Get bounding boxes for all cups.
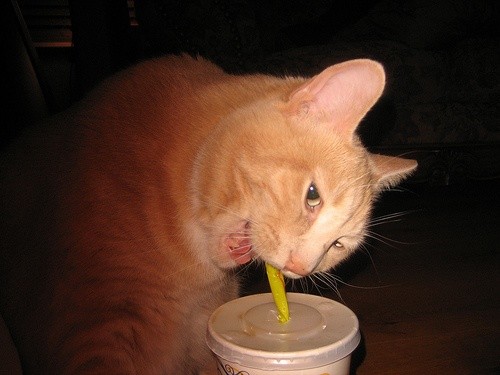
[206,292,361,375]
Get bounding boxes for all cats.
[0,53,419,375]
